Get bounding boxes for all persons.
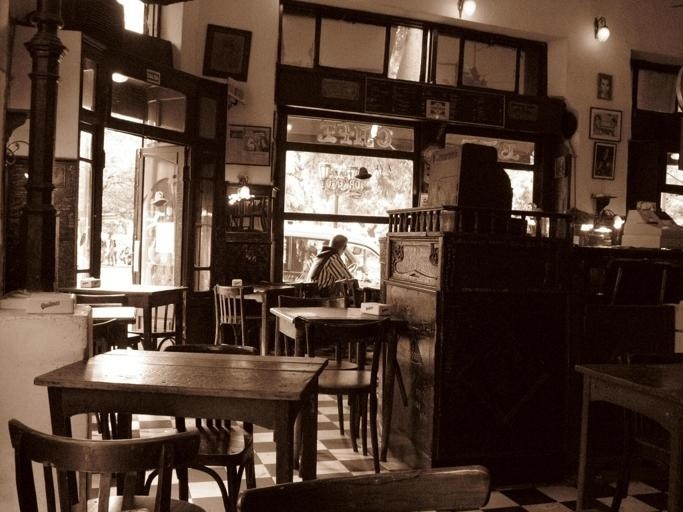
[79,232,132,267]
[594,114,616,137]
[309,235,354,305]
[598,76,613,100]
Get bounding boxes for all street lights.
[317,159,363,229]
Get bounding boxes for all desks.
[33,350,329,495]
[571,358,682,511]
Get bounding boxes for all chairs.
[2,280,408,474]
[144,346,256,511]
[9,418,204,511]
[235,465,491,511]
[578,252,673,511]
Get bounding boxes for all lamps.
[456,1,477,21]
[593,15,611,42]
[577,196,625,237]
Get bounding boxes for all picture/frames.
[586,105,623,144]
[201,22,253,84]
[590,138,616,182]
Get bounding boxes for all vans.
[282,224,381,286]
